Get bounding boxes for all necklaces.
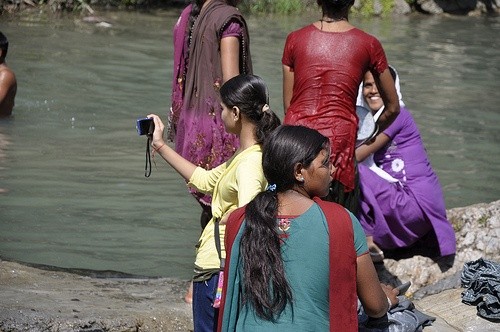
[278,197,304,207]
[320,18,347,30]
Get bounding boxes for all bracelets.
[385,296,391,312]
[156,143,164,151]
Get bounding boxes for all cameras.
[136,117,155,136]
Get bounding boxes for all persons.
[168,0,253,302]
[354,65,456,259]
[282,0,400,220]
[146,74,282,332]
[0,30,16,118]
[216,124,400,332]
